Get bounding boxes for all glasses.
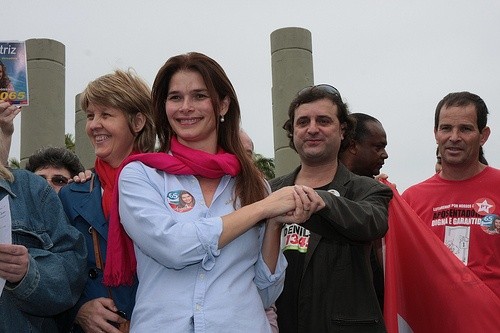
[37,174,69,186]
[296,84,343,102]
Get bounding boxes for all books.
[0,40,29,106]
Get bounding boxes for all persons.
[24,52,307,333]
[178,190,195,208]
[487,216,500,233]
[266,84,500,333]
[0,59,15,105]
[0,99,88,333]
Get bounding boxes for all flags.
[379,177,500,333]
